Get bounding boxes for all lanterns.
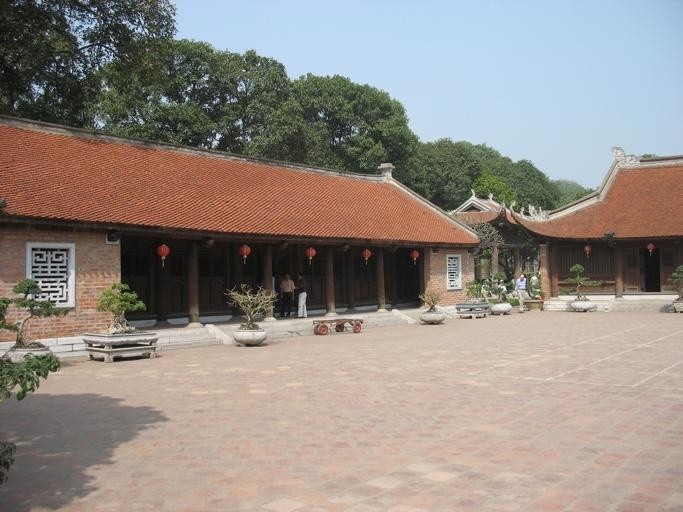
[238,244,251,264]
[410,250,419,265]
[583,245,591,257]
[156,243,169,268]
[304,247,316,266]
[361,248,371,265]
[646,242,654,256]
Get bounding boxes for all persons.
[529,274,538,297]
[292,272,308,318]
[480,278,493,304]
[514,274,528,312]
[278,272,295,317]
[488,274,516,303]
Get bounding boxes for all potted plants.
[0,279,158,366]
[418,287,447,324]
[454,272,546,318]
[662,264,683,314]
[557,262,606,313]
[222,282,280,348]
